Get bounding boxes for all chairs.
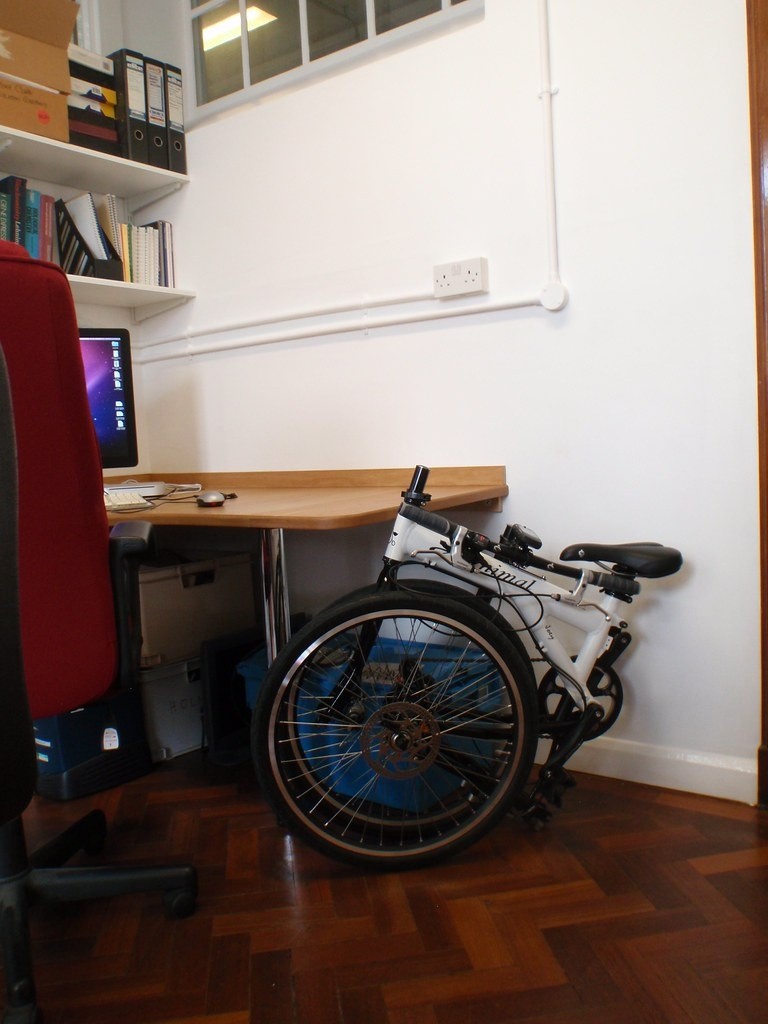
[0,238,198,1024]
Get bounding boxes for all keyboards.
[102,491,155,511]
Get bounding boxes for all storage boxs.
[0,0,79,142]
[140,657,212,761]
[139,546,254,669]
[238,633,499,813]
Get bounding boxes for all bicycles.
[251,467,685,872]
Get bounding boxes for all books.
[0,41,190,290]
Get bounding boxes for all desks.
[97,466,509,783]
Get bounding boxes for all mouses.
[197,492,225,507]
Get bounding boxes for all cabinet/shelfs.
[0,124,194,324]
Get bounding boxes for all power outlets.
[432,258,458,299]
[458,260,489,298]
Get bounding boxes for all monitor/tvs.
[78,328,138,469]
